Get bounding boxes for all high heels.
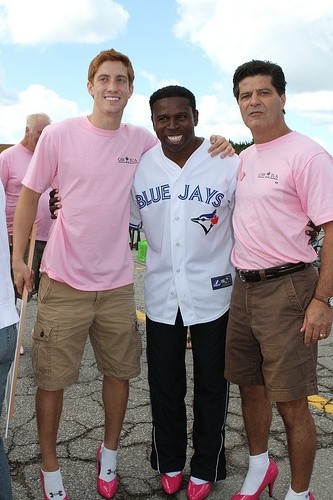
[96,443,118,498]
[39,469,68,500]
[308,491,315,500]
[231,456,278,500]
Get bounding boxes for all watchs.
[313,295,333,308]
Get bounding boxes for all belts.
[235,262,305,282]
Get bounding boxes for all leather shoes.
[187,478,211,500]
[162,473,182,495]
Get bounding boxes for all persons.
[12,48,236,500]
[224,60,333,500]
[0,177,19,500]
[0,114,55,354]
[49,86,321,500]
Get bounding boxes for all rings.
[319,334,326,338]
